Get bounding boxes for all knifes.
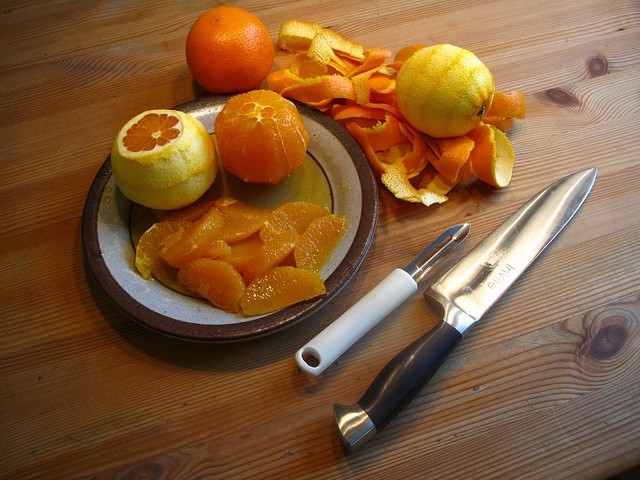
[334,165,598,455]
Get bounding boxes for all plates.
[83,91,377,343]
